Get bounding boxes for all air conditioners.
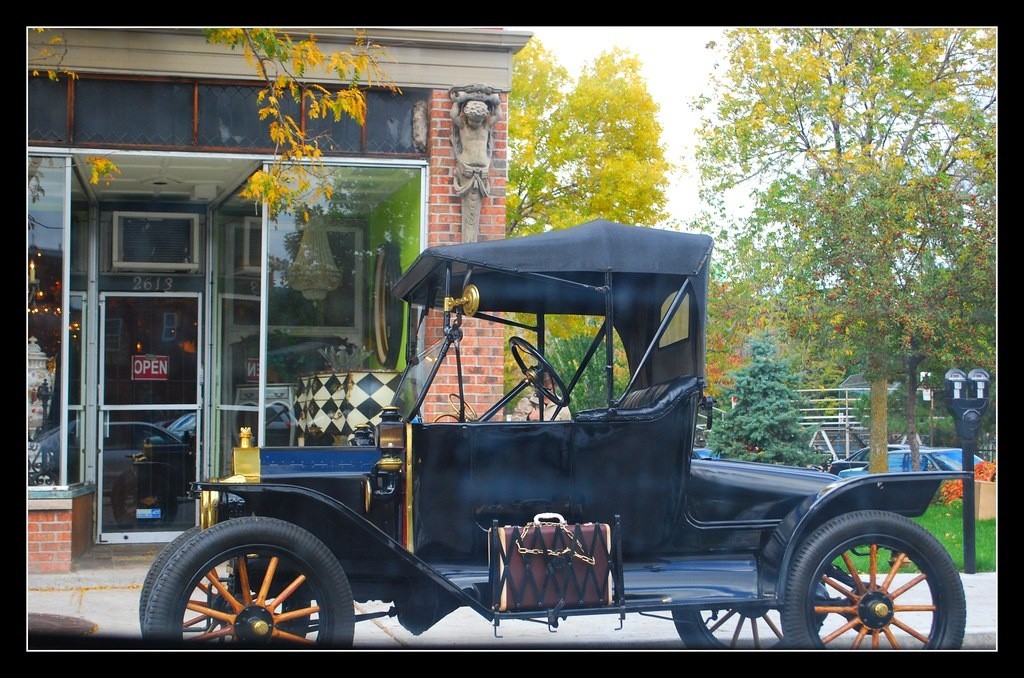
[224,215,262,276]
[111,211,199,274]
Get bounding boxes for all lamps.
[291,212,340,307]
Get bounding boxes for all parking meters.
[944,368,991,575]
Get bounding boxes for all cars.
[26,420,198,531]
[838,447,987,480]
[692,447,720,459]
[827,442,928,477]
[145,405,300,452]
[136,219,967,648]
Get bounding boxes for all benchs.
[572,374,699,422]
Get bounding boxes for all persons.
[510,364,571,421]
